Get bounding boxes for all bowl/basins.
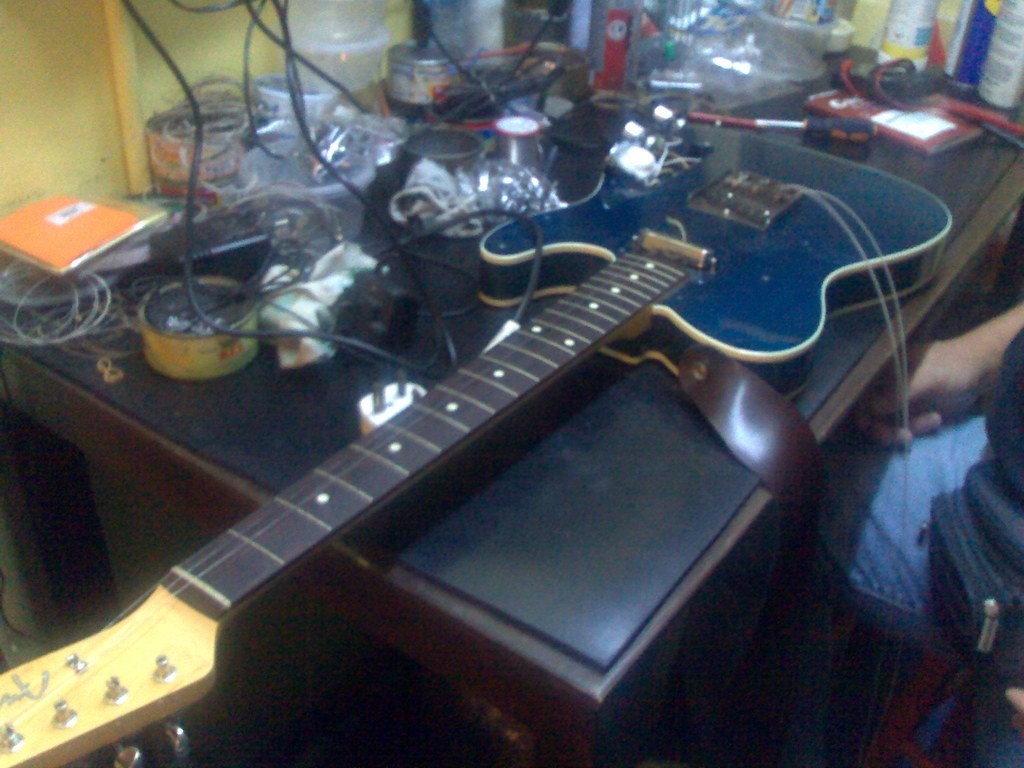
[280,0,391,92]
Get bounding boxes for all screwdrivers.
[756,111,877,145]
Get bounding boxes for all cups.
[406,129,483,181]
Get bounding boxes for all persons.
[699,308,1024,737]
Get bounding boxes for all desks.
[0,74,1021,768]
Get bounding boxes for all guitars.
[2,127,955,767]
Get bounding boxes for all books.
[0,193,168,275]
[805,88,985,157]
